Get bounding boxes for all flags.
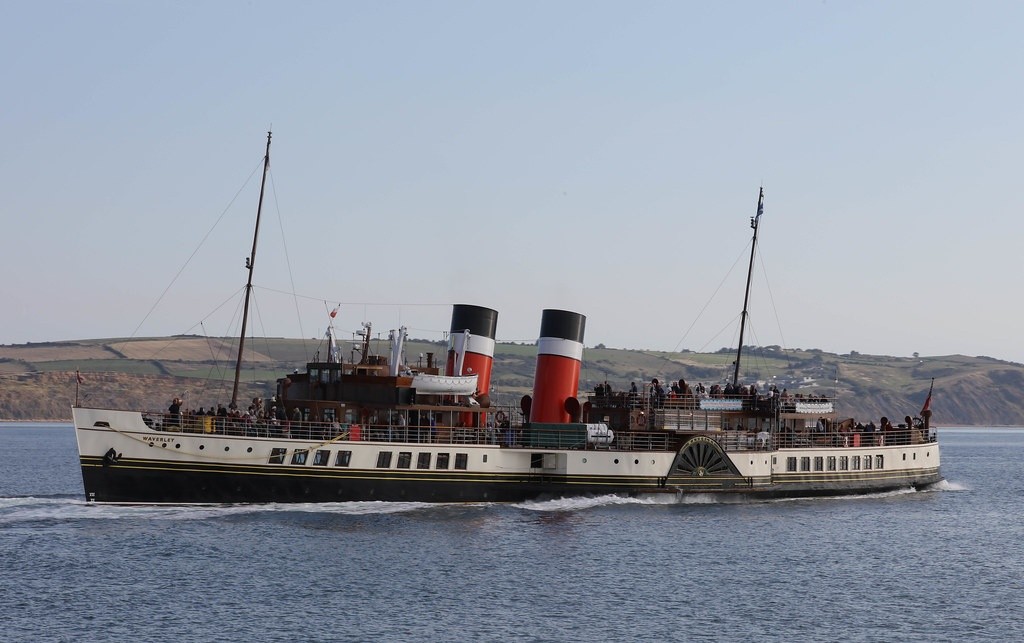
[756,192,765,217]
[919,388,932,417]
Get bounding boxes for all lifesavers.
[637,413,646,426]
[496,411,505,423]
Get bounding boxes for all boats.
[396,366,479,397]
[70,122,947,510]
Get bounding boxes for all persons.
[168,398,180,432]
[594,379,829,409]
[292,407,302,439]
[314,415,348,440]
[838,421,876,447]
[816,419,824,432]
[184,404,282,437]
[397,414,405,439]
[495,416,512,448]
[420,414,429,444]
